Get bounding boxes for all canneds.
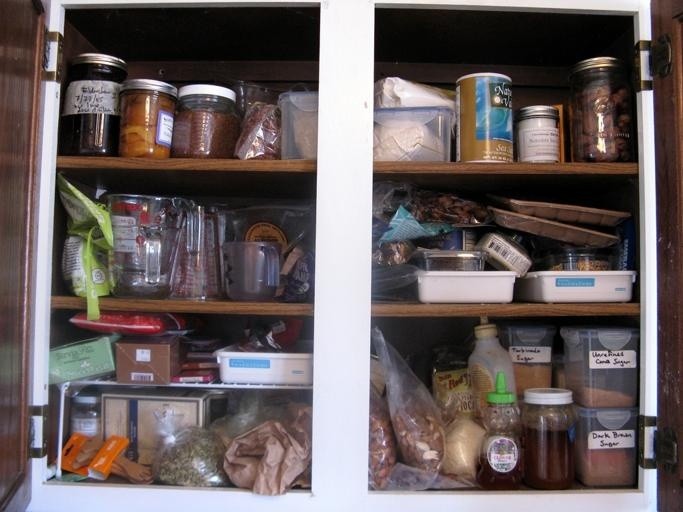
[455,71,514,165]
[58,53,127,157]
[70,395,102,439]
[521,387,576,490]
[117,78,177,161]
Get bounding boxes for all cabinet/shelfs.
[31,0,657,511]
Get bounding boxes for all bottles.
[467,324,518,426]
[171,204,221,297]
[477,370,529,489]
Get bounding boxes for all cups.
[220,237,284,302]
[102,192,199,300]
